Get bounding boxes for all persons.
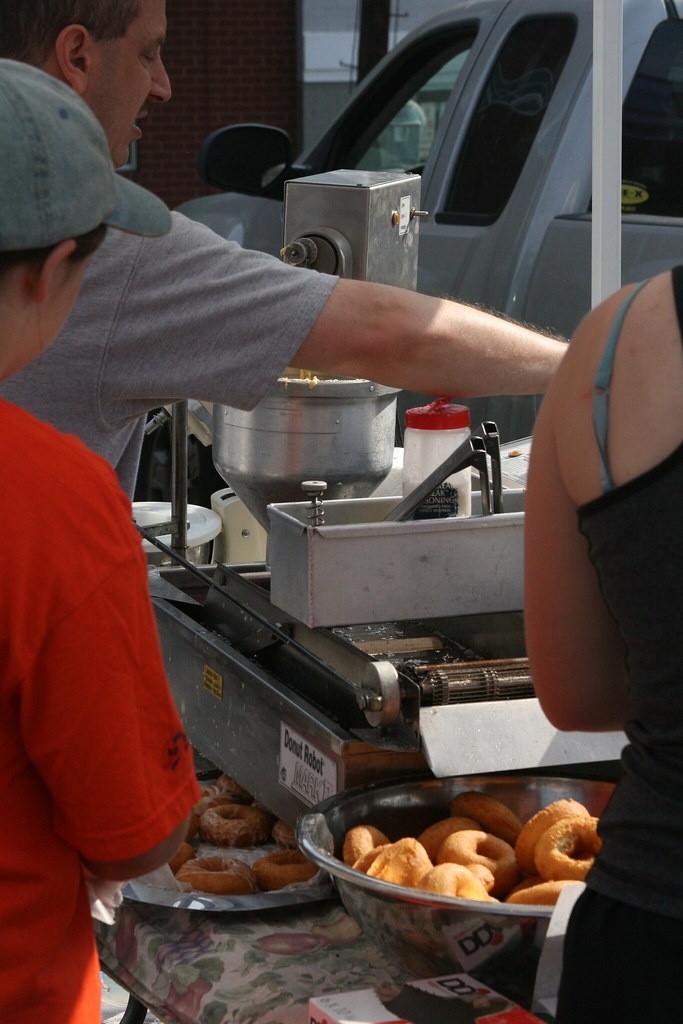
[0,57,200,1024]
[0,0,568,503]
[523,263,683,1024]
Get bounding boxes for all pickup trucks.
[133,0,683,506]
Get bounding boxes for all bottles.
[402,398,472,520]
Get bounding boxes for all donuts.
[169,774,319,896]
[344,792,599,906]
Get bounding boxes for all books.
[308,973,546,1024]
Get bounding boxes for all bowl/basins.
[293,774,615,982]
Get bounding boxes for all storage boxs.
[307,977,550,1024]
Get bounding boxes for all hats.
[0,57,173,251]
